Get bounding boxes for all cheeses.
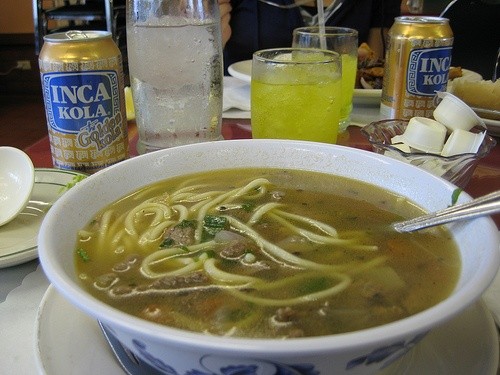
[451,77,500,110]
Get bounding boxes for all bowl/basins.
[36,137,500,375]
[360,118,496,191]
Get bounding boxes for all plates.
[470,106,500,135]
[225,57,484,105]
[0,166,92,269]
[36,282,500,375]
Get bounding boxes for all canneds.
[39,30,130,174]
[380,16,452,120]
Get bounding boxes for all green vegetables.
[80,185,358,321]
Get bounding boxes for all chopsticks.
[395,188,500,231]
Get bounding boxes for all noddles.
[77,167,461,339]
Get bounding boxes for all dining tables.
[0,85,500,375]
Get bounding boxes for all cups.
[291,26,358,134]
[125,1,225,154]
[248,47,341,144]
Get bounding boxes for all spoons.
[0,146,34,226]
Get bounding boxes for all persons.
[217,0,403,76]
